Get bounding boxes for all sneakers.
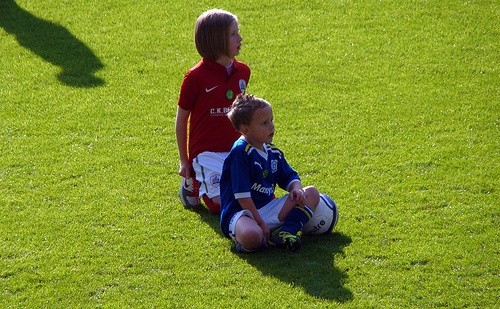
[271,230,302,252]
[179,175,200,207]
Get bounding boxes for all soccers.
[301,194,340,235]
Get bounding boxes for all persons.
[218,95,319,253]
[175,9,275,214]
